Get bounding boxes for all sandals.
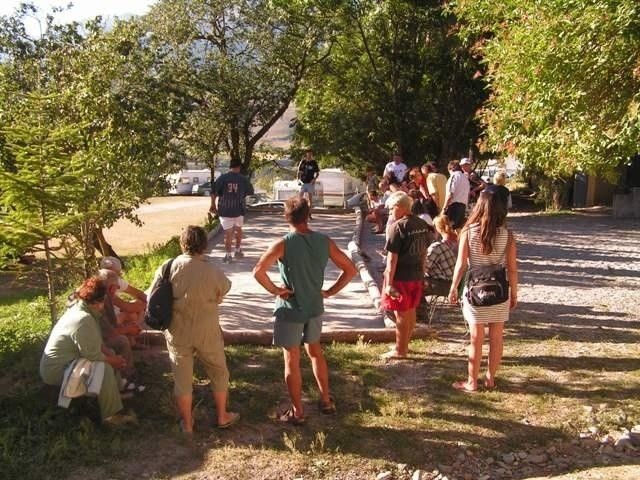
[119,381,145,392]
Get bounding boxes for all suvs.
[474,168,493,186]
[246,193,272,205]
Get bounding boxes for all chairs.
[427,262,470,340]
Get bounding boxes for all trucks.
[167,169,222,196]
[273,181,323,206]
[312,169,352,207]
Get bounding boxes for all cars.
[190,181,216,196]
[503,168,516,179]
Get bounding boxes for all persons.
[297,150,320,220]
[209,158,254,263]
[252,197,357,425]
[365,153,516,394]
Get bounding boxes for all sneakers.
[321,394,337,414]
[224,256,231,263]
[235,249,243,256]
[280,408,304,424]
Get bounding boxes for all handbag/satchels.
[144,278,172,329]
[465,264,509,305]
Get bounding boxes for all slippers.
[453,382,477,393]
[218,411,241,429]
[483,379,498,392]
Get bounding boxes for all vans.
[248,200,286,215]
